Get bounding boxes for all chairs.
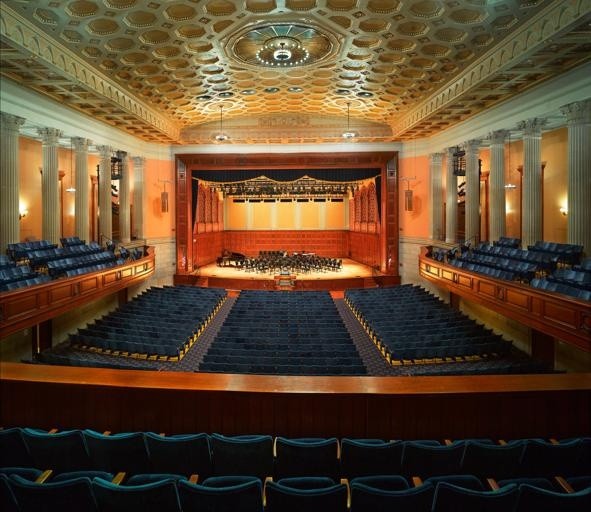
[433,236,591,306]
[2,236,142,294]
[24,283,564,374]
[0,418,591,512]
[216,250,342,274]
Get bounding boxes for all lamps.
[208,102,236,142]
[254,39,311,70]
[159,178,169,214]
[398,176,418,212]
[336,100,363,138]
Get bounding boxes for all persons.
[433,243,470,264]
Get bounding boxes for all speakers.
[405,190,413,211]
[161,192,168,212]
